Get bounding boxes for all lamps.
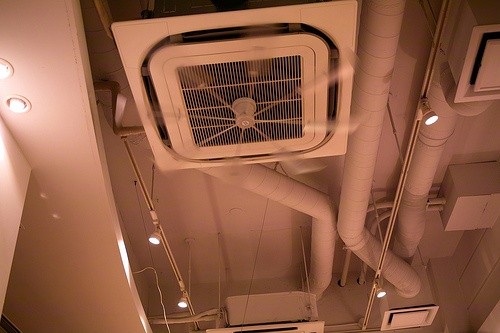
[148,225,162,245]
[177,293,189,308]
[375,279,386,298]
[420,100,438,126]
